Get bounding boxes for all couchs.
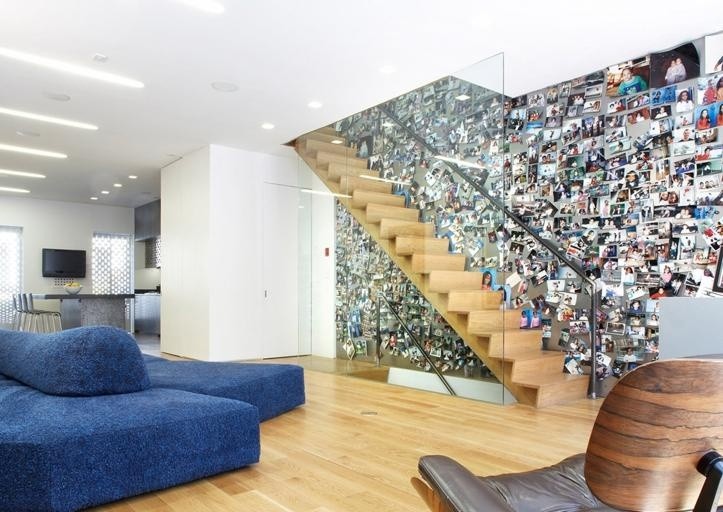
[0,324,305,512]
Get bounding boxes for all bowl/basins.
[63,285,83,294]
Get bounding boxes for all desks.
[32,293,136,331]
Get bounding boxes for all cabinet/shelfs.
[134,199,160,241]
[135,295,160,335]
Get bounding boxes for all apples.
[65,282,80,286]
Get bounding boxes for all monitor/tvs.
[42,248,87,278]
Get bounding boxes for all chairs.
[10,293,62,334]
[417,358,722,511]
